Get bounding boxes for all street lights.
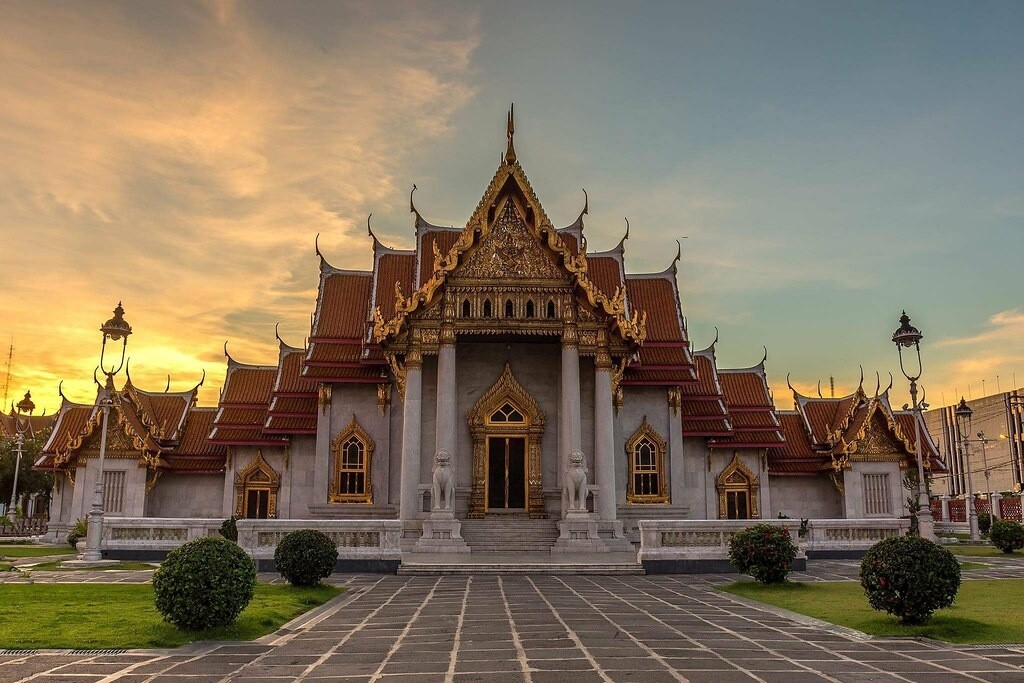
[82,300,133,562]
[5,389,36,535]
[891,309,935,544]
[954,396,981,539]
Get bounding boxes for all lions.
[565,449,588,511]
[432,448,456,511]
